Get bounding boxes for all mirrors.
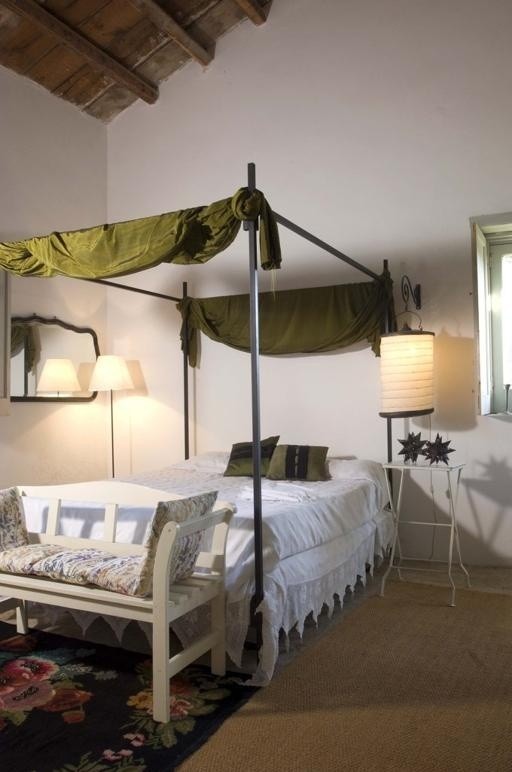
[11,314,99,403]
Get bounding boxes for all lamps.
[89,355,134,478]
[378,273,435,417]
[36,358,83,397]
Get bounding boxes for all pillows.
[223,436,331,483]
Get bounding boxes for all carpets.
[0,618,268,771]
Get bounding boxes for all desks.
[380,460,473,608]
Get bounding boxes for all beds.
[1,454,397,668]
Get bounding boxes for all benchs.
[1,480,237,726]
